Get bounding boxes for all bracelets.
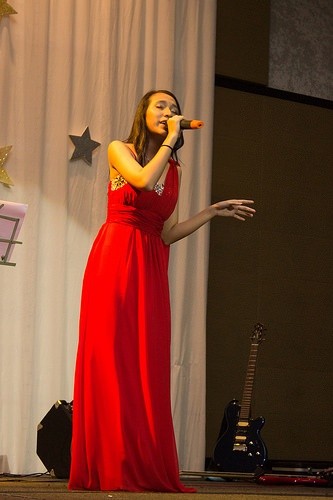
[162,145,173,150]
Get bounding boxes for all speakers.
[36,399,73,479]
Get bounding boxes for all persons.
[68,90,256,492]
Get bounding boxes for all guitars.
[213,322,266,482]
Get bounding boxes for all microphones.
[180,119,204,129]
